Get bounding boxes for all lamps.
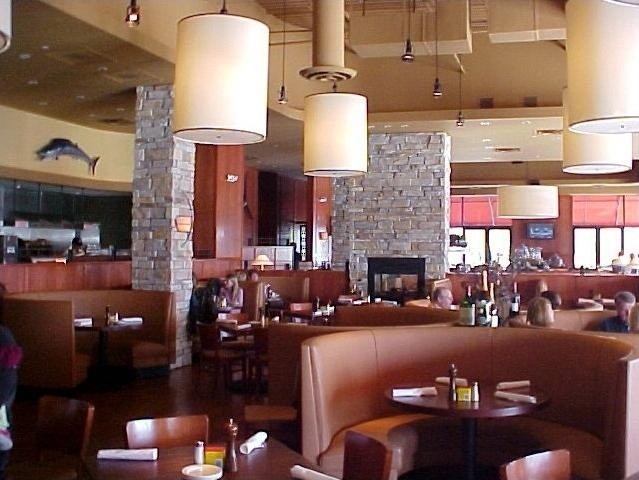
[304,82,368,177]
[432,0,443,96]
[565,0,639,134]
[124,0,141,28]
[0,0,12,54]
[498,162,559,220]
[563,87,633,174]
[278,0,288,104]
[401,0,416,63]
[173,0,270,144]
[251,255,274,271]
[457,54,464,126]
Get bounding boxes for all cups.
[374,298,381,303]
[218,296,226,308]
[218,312,227,321]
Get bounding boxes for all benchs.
[190,269,638,397]
[3,285,185,391]
[295,322,639,480]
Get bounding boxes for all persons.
[187,270,259,337]
[68,238,82,259]
[427,287,454,311]
[525,252,639,333]
[0,327,24,480]
[290,242,302,270]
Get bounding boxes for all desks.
[71,315,147,379]
[77,434,328,480]
[382,377,549,480]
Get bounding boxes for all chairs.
[123,413,214,450]
[497,446,571,479]
[342,429,400,480]
[2,394,96,479]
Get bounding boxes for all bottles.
[109,245,117,258]
[192,440,205,464]
[348,249,369,297]
[510,242,565,274]
[459,269,521,327]
[446,362,458,404]
[470,381,479,402]
[623,253,639,276]
[105,305,111,320]
[612,251,624,275]
[220,415,238,472]
